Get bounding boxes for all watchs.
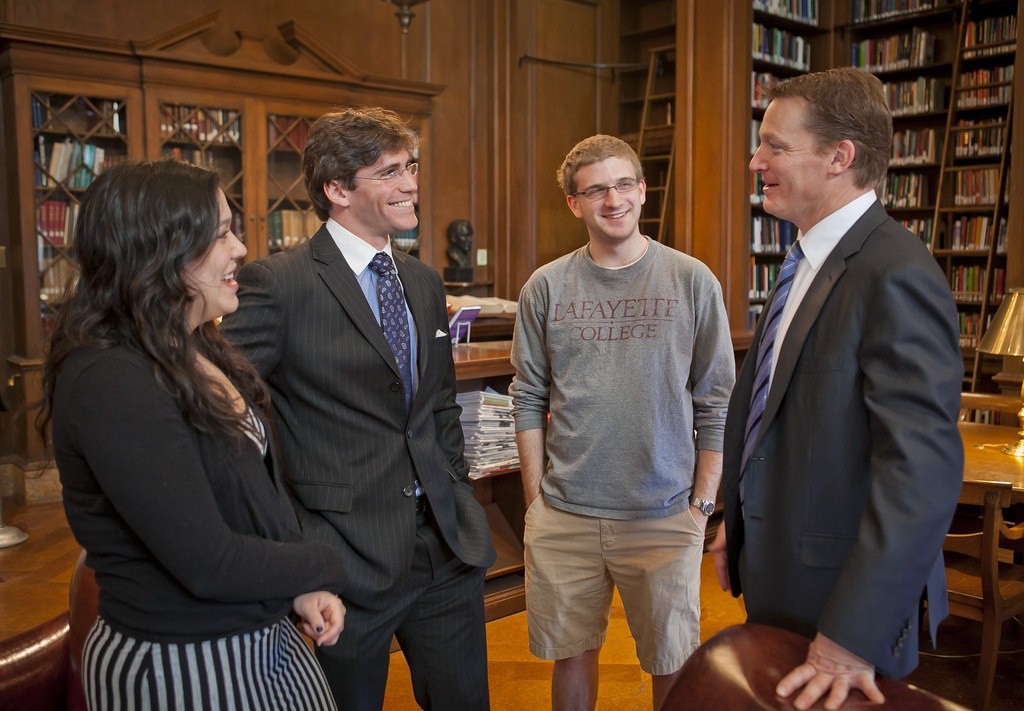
[690,497,716,516]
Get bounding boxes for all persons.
[217,106,498,711]
[445,220,474,268]
[710,66,965,710]
[510,134,735,711]
[51,158,346,711]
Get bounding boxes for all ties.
[739,240,803,519]
[369,253,412,411]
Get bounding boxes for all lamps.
[976,288,1024,457]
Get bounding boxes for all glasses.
[335,163,418,187]
[571,180,638,199]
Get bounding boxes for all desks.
[957,421,1024,505]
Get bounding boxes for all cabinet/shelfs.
[451,340,527,623]
[747,0,1024,373]
[620,23,674,247]
[0,43,434,461]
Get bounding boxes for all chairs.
[924,479,1024,711]
[961,393,1022,425]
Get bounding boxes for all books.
[33,94,328,339]
[449,306,482,345]
[456,386,550,481]
[849,0,1020,422]
[391,227,418,247]
[749,0,818,332]
